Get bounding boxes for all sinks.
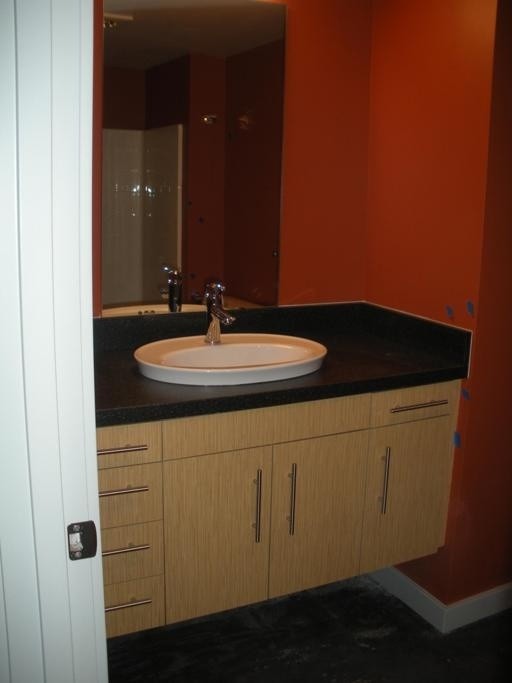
[133,332,327,385]
[102,304,208,318]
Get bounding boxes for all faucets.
[161,265,183,313]
[205,282,236,344]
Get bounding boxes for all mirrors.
[103,2,289,317]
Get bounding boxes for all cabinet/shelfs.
[160,393,372,625]
[96,420,165,639]
[360,380,460,576]
